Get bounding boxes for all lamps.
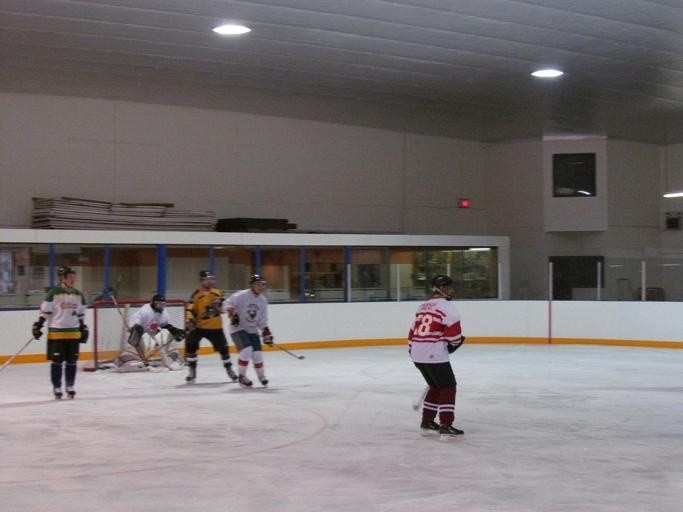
[661,191,683,198]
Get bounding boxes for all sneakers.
[184,367,197,382]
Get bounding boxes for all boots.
[439,424,465,435]
[258,375,268,385]
[419,418,440,433]
[237,375,253,386]
[53,384,63,396]
[65,384,76,395]
[224,367,238,380]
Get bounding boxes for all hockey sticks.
[413,388,428,410]
[169,313,218,342]
[109,294,169,373]
[259,334,303,360]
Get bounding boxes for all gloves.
[262,336,274,345]
[228,314,240,327]
[79,325,90,344]
[164,325,185,342]
[32,322,44,341]
[127,322,143,347]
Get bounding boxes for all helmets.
[152,296,165,307]
[58,262,76,280]
[432,275,453,294]
[199,270,216,280]
[248,273,267,285]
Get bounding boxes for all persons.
[95,287,114,303]
[222,273,273,386]
[407,274,465,435]
[31,265,90,396]
[184,270,238,381]
[113,294,184,373]
